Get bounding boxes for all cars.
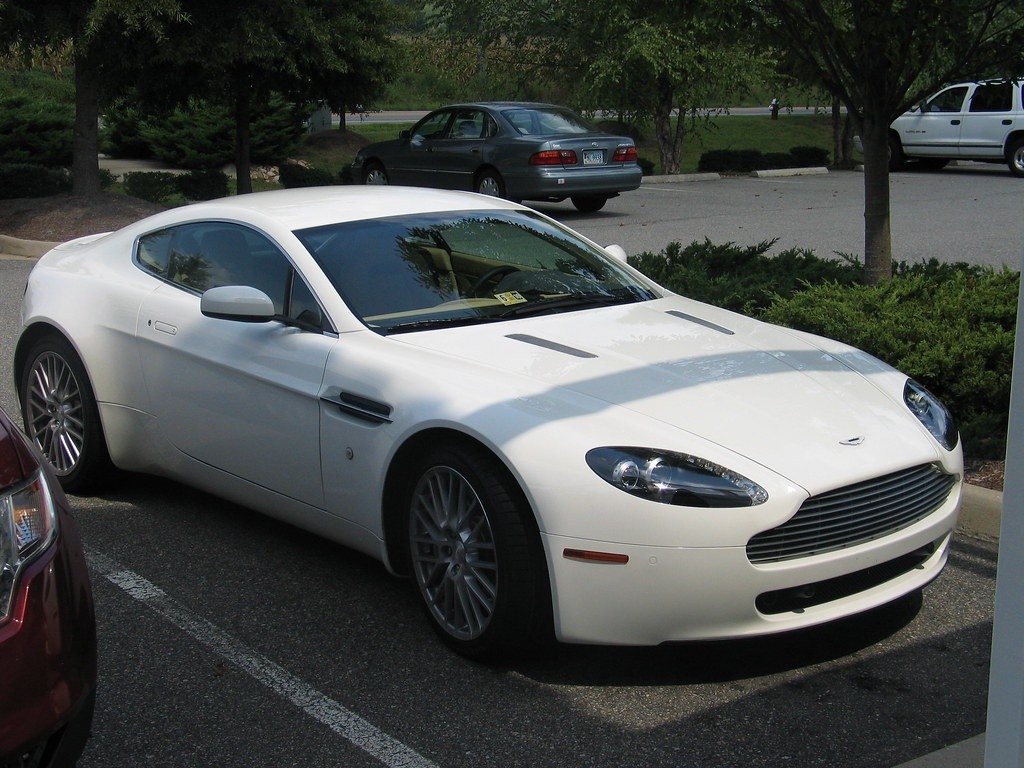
[885,77,1024,177]
[0,407,99,768]
[350,101,643,211]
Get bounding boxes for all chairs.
[234,251,322,328]
[201,229,249,291]
[456,121,480,138]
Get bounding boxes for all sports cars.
[13,186,964,670]
